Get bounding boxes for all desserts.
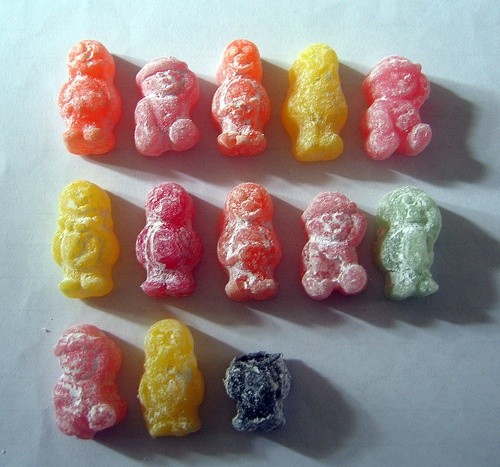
[211,38,270,157]
[282,43,348,162]
[216,181,284,300]
[225,349,291,434]
[374,188,443,300]
[138,317,204,437]
[59,40,120,154]
[134,56,201,156]
[361,56,432,161]
[51,180,119,298]
[135,182,202,298]
[52,323,128,439]
[300,190,368,300]
